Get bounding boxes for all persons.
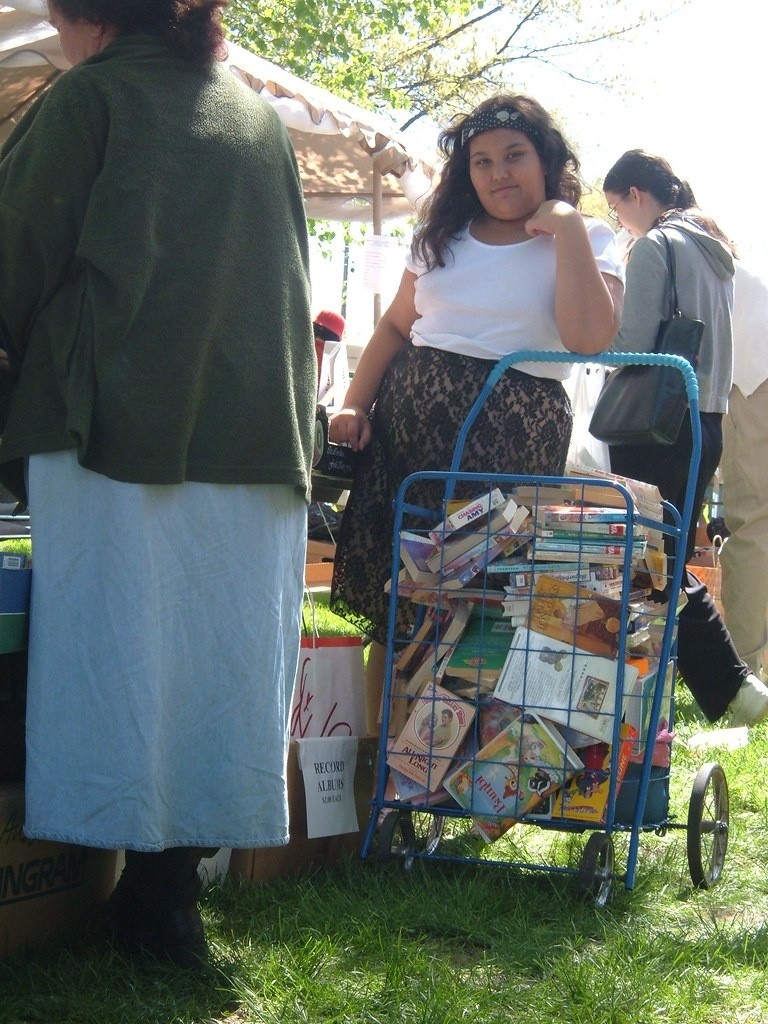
[0,0,345,991]
[418,715,441,745]
[326,93,767,735]
[434,709,454,743]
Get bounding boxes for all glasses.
[607,188,647,221]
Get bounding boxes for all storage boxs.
[225,738,377,888]
[0,783,127,963]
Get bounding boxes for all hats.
[313,310,346,342]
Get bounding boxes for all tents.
[1,0,450,343]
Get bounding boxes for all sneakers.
[720,674,768,728]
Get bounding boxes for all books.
[384,465,670,821]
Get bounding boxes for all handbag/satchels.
[289,582,368,743]
[588,228,706,446]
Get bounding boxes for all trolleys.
[365,349,734,911]
